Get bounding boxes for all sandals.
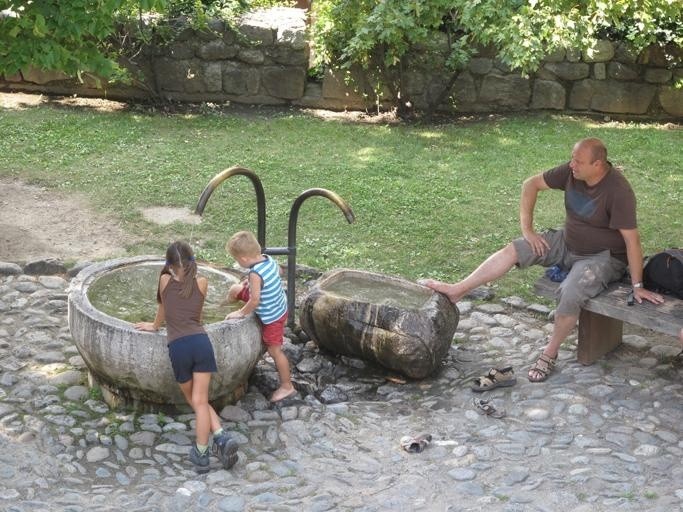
[471,366,516,392]
[528,353,558,383]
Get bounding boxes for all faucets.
[196,164,357,331]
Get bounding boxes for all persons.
[133,240,239,470]
[415,138,665,382]
[220,231,294,402]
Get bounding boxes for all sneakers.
[189,442,209,472]
[212,432,239,469]
[401,432,432,453]
[474,398,502,418]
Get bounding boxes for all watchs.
[632,282,645,289]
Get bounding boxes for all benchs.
[534,275,683,366]
